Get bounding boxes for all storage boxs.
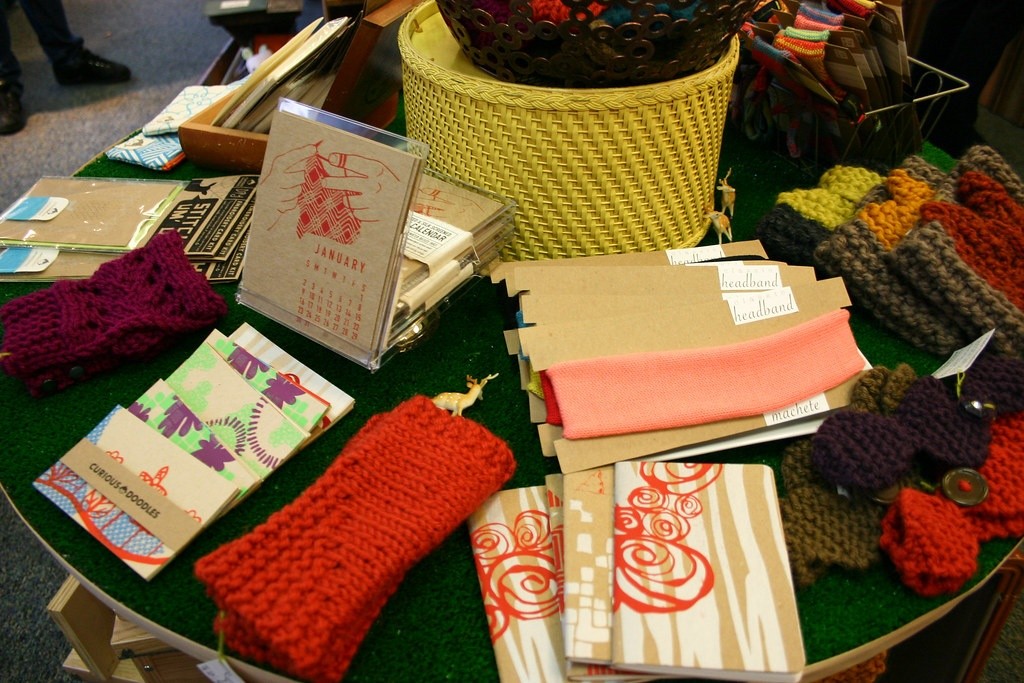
[178,1,429,173]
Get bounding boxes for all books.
[31,322,355,584]
[468,463,804,683]
[211,14,362,133]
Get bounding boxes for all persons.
[0,0,133,137]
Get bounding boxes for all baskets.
[733,47,969,190]
[438,0,752,87]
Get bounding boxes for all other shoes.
[0,80,26,135]
[53,48,131,86]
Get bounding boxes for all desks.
[0,81,1024,683]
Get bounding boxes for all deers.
[431,372,499,417]
[716,167,736,218]
[703,211,732,244]
